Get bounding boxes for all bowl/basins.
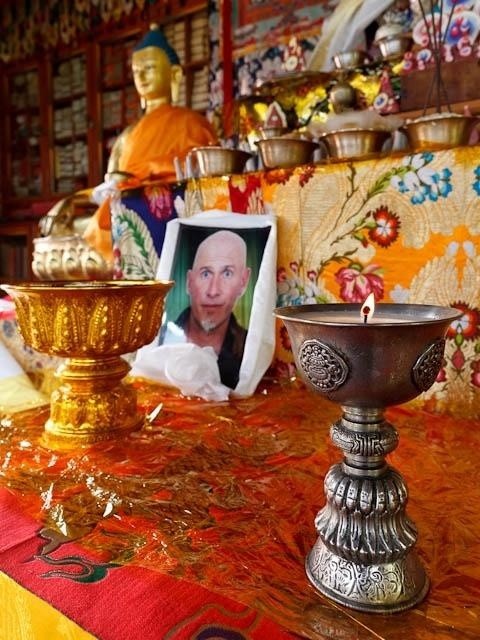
[188,113,480,178]
[332,34,415,68]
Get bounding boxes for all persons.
[68,26,220,271]
[159,224,257,395]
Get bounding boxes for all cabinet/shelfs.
[0,1,212,282]
